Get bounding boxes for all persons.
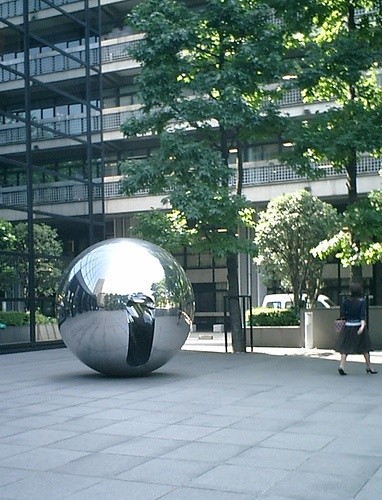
[331,281,378,375]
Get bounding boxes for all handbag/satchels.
[334,317,346,335]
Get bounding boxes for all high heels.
[366,367,378,374]
[338,366,347,376]
